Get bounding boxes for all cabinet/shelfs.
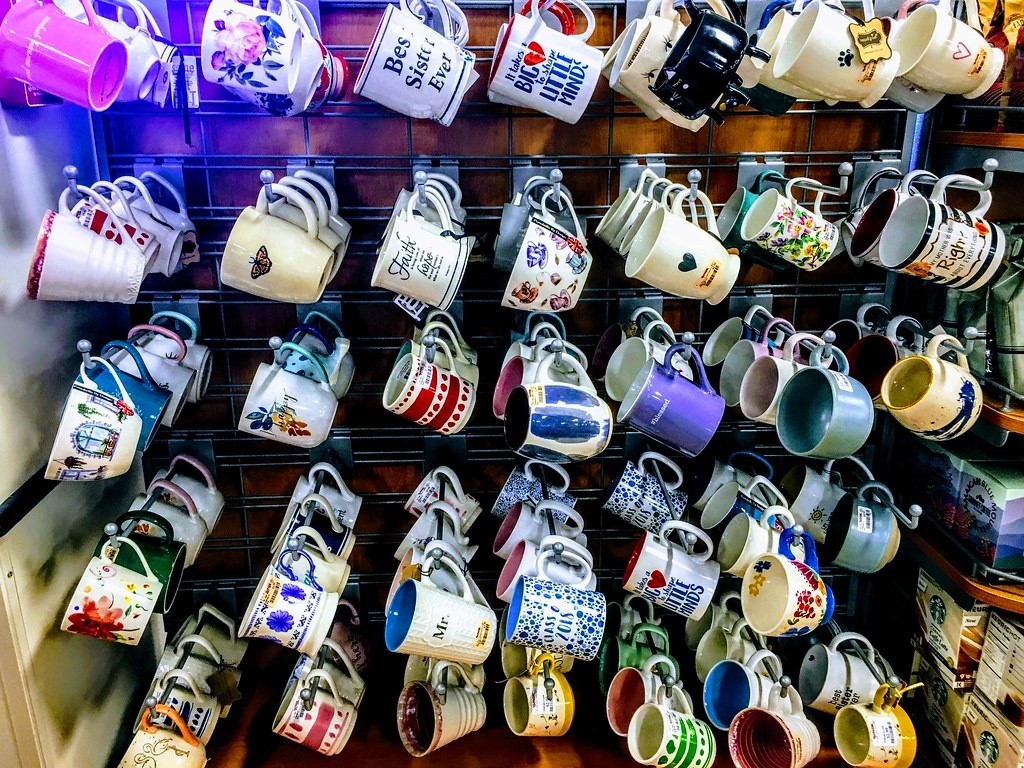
[898,101,1024,614]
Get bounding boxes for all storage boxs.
[900,563,1024,768]
[908,434,1024,586]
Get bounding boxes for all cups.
[353,0,480,127]
[588,304,983,459]
[238,462,368,756]
[370,173,476,311]
[601,0,771,132]
[0,0,180,112]
[26,171,200,304]
[220,170,352,303]
[494,176,593,311]
[487,0,603,125]
[493,310,614,464]
[385,465,497,758]
[595,168,740,305]
[841,167,1005,292]
[116,603,248,768]
[602,452,917,768]
[491,459,607,736]
[716,170,839,271]
[382,311,480,435]
[201,0,349,117]
[60,455,224,645]
[43,310,212,480]
[750,0,1004,116]
[238,312,355,448]
